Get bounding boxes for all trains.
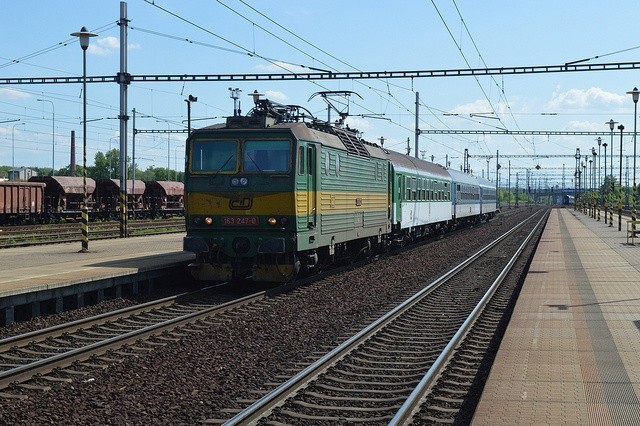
[2,173,184,224]
[181,90,496,288]
